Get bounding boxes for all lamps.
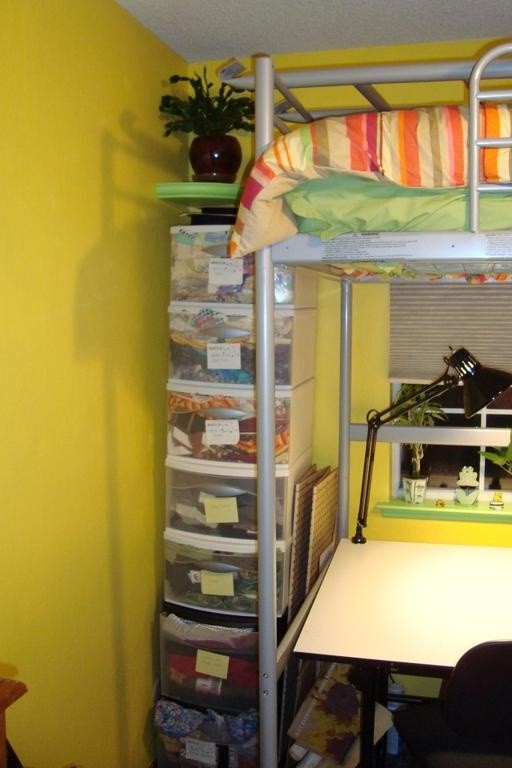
[419,632,511,767]
[352,345,511,543]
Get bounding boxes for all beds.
[223,35,512,274]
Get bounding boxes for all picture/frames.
[294,537,510,766]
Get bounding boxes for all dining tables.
[157,65,258,182]
[393,384,450,503]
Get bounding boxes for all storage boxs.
[154,224,321,766]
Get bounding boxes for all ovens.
[223,35,512,274]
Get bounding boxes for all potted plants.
[157,65,258,182]
[393,384,450,503]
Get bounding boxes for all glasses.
[352,345,511,543]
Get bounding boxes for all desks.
[294,537,510,766]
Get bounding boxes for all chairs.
[419,632,511,767]
